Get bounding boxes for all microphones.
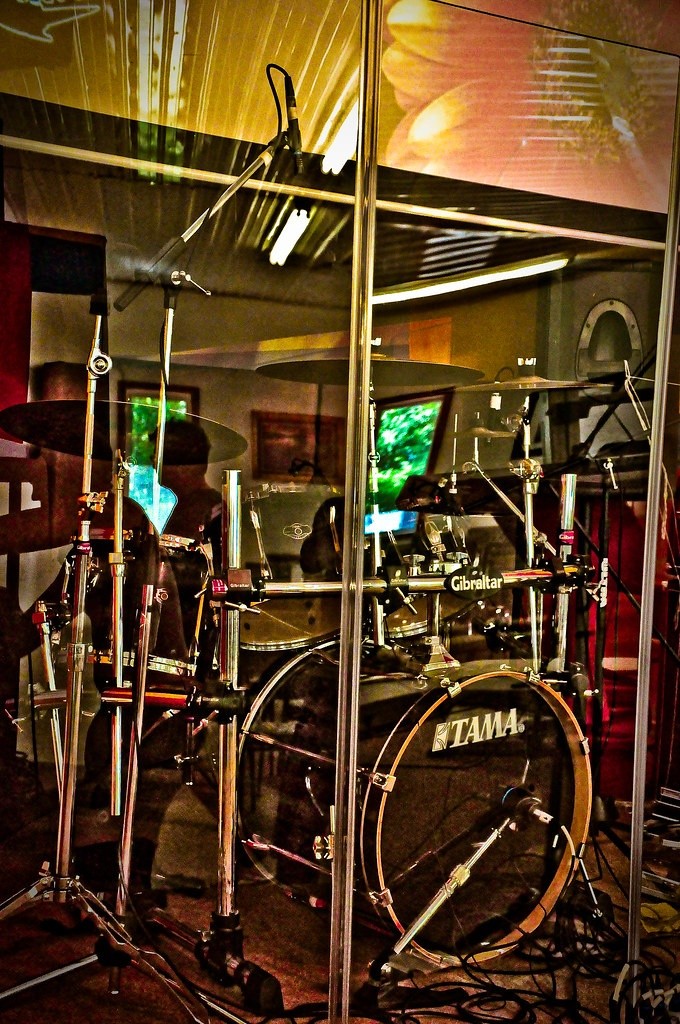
[500,785,561,827]
[284,75,305,175]
[485,377,501,447]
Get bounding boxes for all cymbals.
[450,426,518,440]
[0,398,249,466]
[255,354,486,386]
[452,365,614,391]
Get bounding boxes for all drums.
[359,474,487,639]
[54,527,213,680]
[235,636,593,968]
[204,481,346,653]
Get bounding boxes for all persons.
[0,417,227,937]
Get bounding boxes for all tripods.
[585,462,680,909]
[0,131,289,1024]
